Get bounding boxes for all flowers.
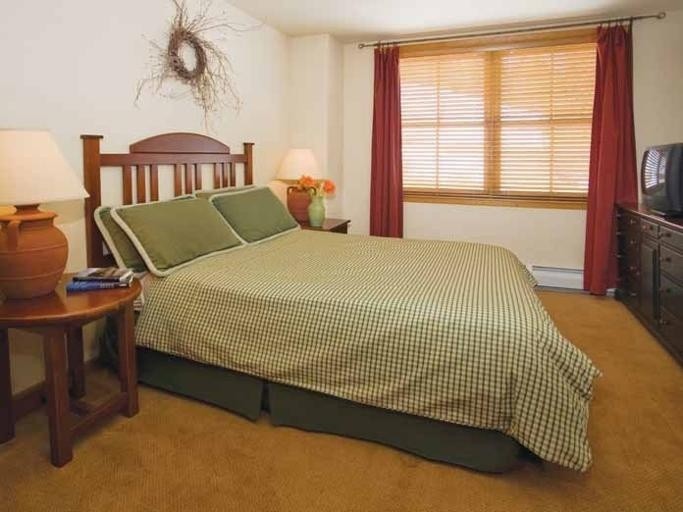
[298,175,335,196]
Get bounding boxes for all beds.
[80,132,601,478]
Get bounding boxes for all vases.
[306,194,326,227]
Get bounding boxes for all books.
[73,267,134,282]
[66,280,131,295]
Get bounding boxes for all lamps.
[0,132,91,298]
[273,149,324,225]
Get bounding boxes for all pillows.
[110,197,246,277]
[192,185,255,202]
[93,194,195,278]
[209,186,301,246]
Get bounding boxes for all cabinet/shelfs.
[613,203,682,368]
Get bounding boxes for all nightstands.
[299,218,351,233]
[0,273,141,467]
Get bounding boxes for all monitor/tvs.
[640,143,683,217]
